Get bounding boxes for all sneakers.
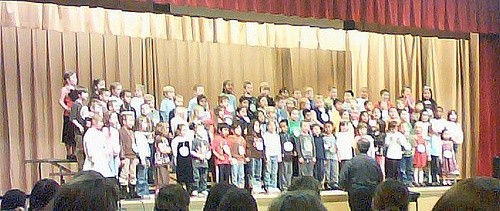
[137,183,283,199]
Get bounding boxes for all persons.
[1,70,500,211]
